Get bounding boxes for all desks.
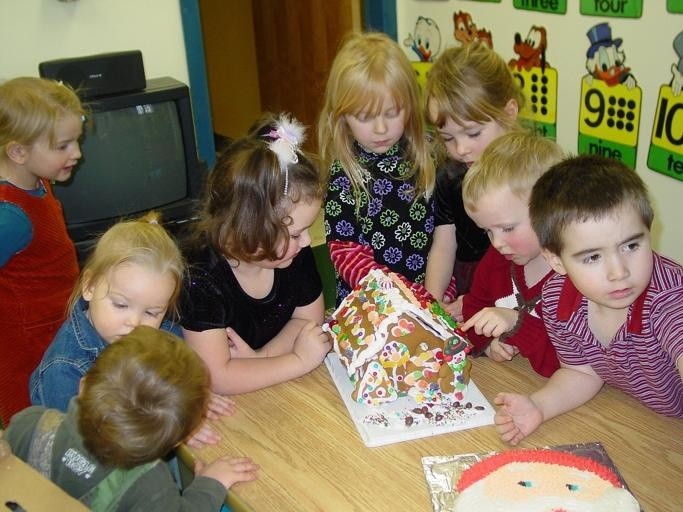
[176,342,682,512]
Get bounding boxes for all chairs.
[0,440,97,511]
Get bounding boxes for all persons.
[30,206,236,447]
[422,38,522,320]
[318,31,458,308]
[0,320,257,511]
[461,130,561,380]
[0,74,86,431]
[493,148,683,448]
[176,112,333,396]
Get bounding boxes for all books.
[420,440,644,512]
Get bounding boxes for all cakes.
[321,268,486,429]
[456,449,641,512]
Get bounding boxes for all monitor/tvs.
[51,77,202,241]
